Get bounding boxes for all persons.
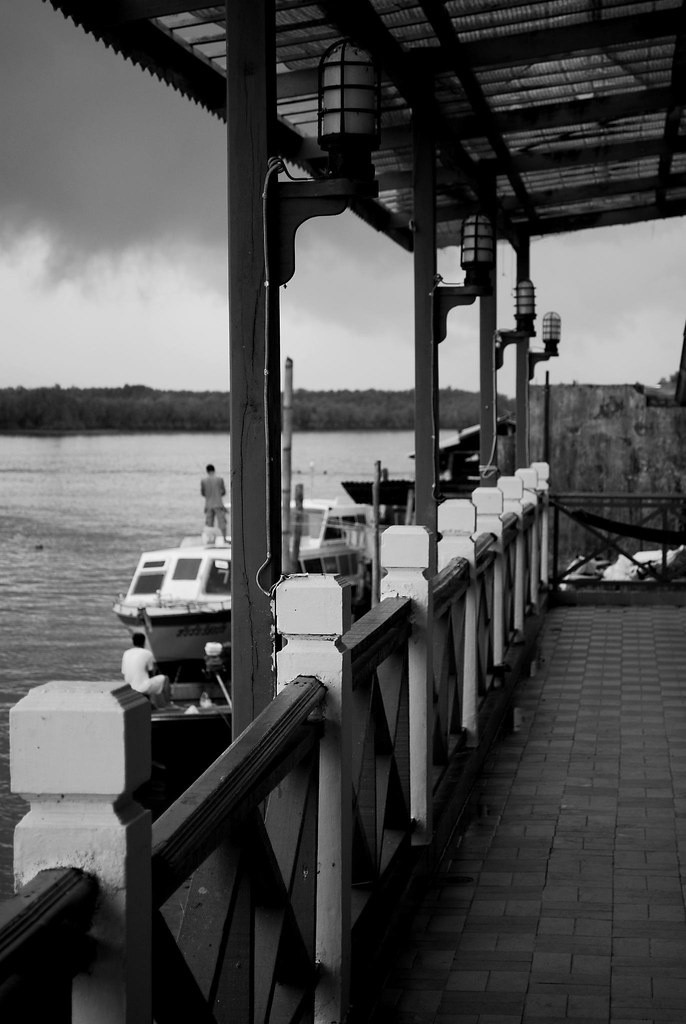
[122,632,180,710]
[201,464,231,543]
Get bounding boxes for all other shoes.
[166,701,181,710]
[149,700,158,711]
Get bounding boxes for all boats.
[106,359,454,787]
